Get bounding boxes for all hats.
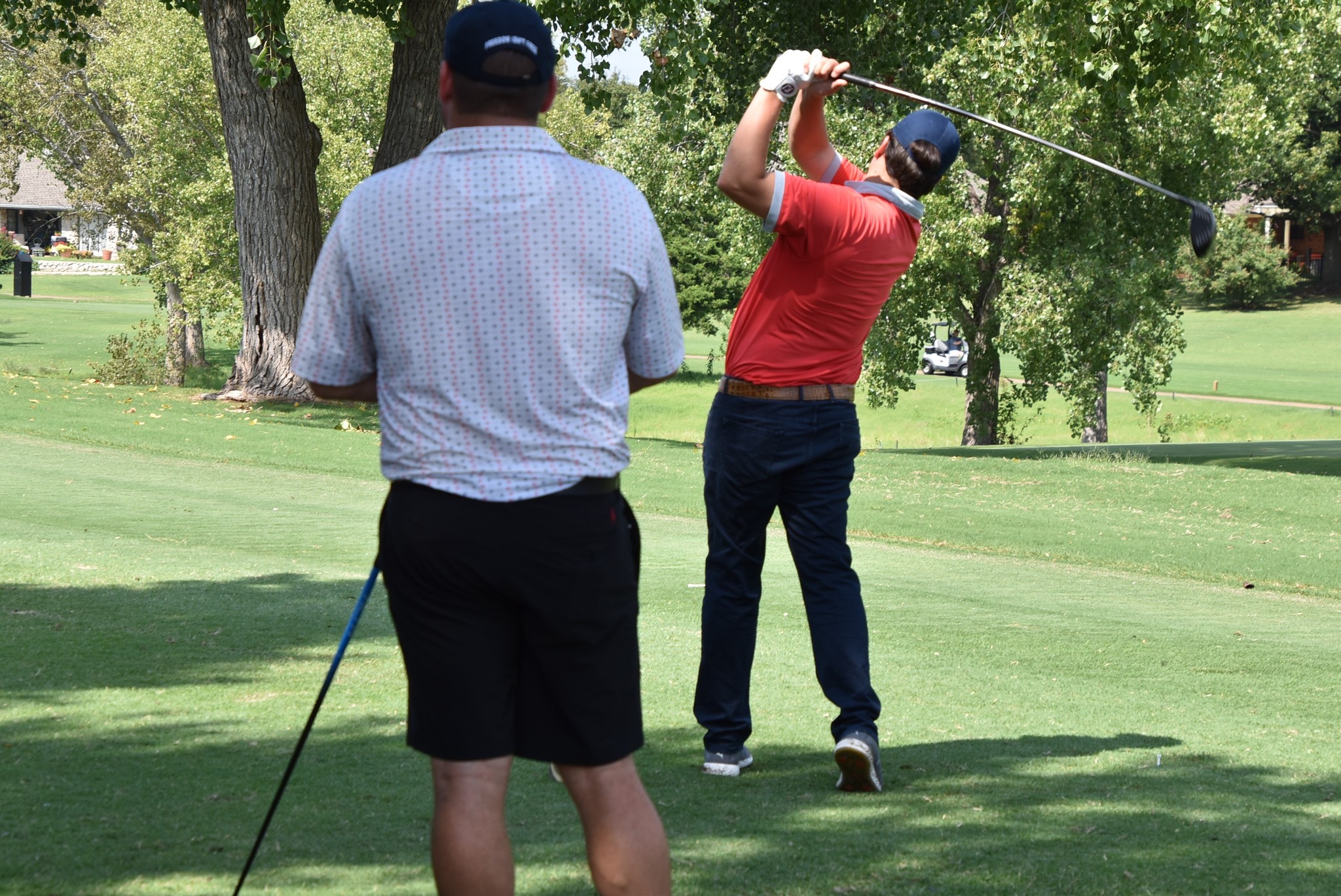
[893,110,959,173]
[444,0,555,87]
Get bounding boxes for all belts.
[717,376,856,401]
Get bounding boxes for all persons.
[691,48,968,792]
[293,2,689,895]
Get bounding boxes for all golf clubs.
[844,72,1218,257]
[233,568,377,896]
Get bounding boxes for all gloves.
[762,47,819,104]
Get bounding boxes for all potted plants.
[54,231,62,236]
[56,244,75,258]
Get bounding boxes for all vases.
[102,250,112,260]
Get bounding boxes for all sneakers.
[832,731,881,791]
[705,748,755,778]
